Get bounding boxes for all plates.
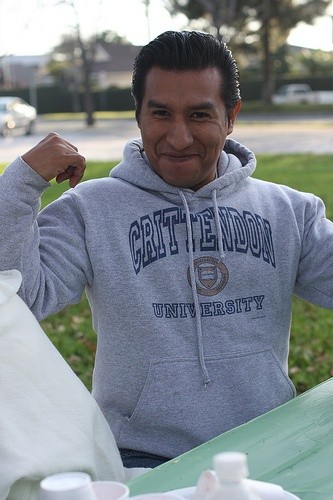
[164,485,300,500]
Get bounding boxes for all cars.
[0,96,37,138]
[269,82,333,107]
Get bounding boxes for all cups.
[38,471,97,500]
[92,481,129,500]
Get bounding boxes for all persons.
[0,30,333,473]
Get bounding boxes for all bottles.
[209,451,260,500]
